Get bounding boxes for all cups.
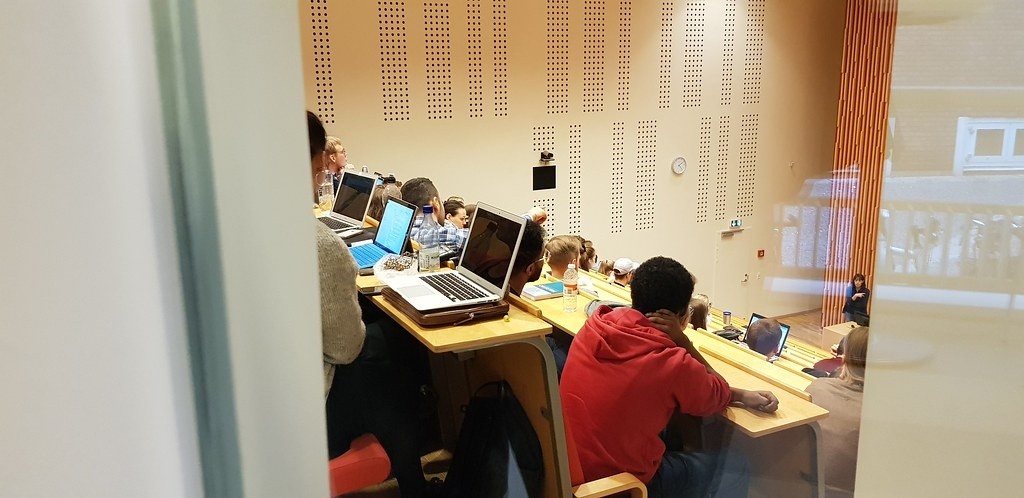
[722,311,731,325]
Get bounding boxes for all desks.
[821,321,862,353]
[314,202,835,498]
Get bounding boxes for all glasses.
[580,244,586,255]
[524,248,552,268]
[329,147,346,155]
[591,255,598,263]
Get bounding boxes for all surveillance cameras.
[543,151,554,158]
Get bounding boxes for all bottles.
[563,263,578,313]
[418,205,440,274]
[362,165,369,173]
[318,170,334,206]
[381,175,403,208]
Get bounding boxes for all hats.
[613,258,640,275]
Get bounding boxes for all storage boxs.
[372,252,418,286]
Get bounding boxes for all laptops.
[348,194,418,268]
[383,201,528,313]
[315,169,381,232]
[739,312,790,361]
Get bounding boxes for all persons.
[372,179,565,378]
[545,236,597,277]
[747,319,782,357]
[804,326,869,491]
[304,112,446,497]
[690,294,711,330]
[325,137,348,194]
[598,258,640,285]
[843,275,869,323]
[559,256,779,498]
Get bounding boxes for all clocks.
[671,156,687,175]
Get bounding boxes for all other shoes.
[400,477,443,498]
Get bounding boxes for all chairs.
[325,431,392,498]
[471,292,649,498]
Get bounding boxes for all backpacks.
[442,379,546,498]
